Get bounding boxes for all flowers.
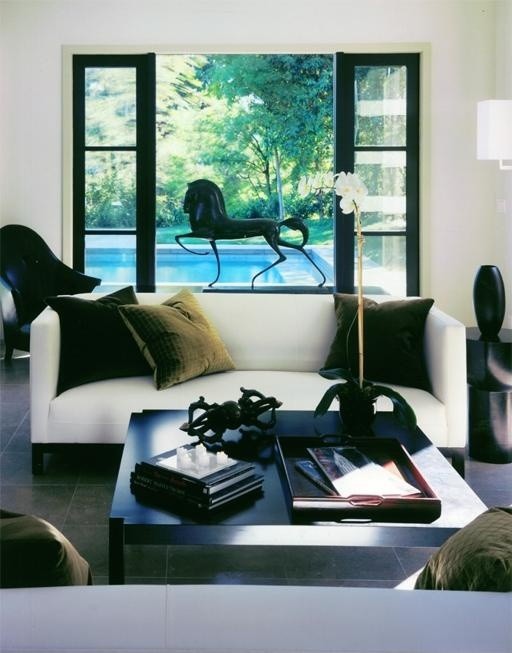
[298,170,369,387]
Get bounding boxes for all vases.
[338,394,374,437]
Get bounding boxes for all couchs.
[29,292,468,481]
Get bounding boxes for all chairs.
[1,223,103,366]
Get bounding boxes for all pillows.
[119,288,236,390]
[46,284,152,396]
[325,292,433,388]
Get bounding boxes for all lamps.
[475,98,512,170]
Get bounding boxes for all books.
[306,442,420,498]
[294,456,339,498]
[129,443,266,512]
